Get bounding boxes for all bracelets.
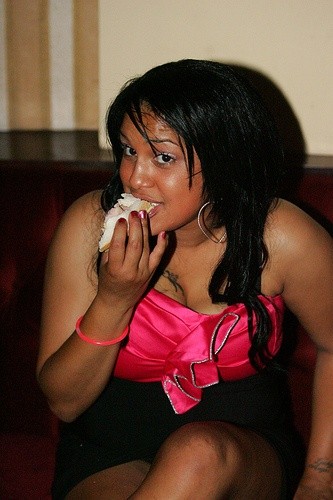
[73,315,130,345]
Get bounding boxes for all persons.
[38,59,333,500]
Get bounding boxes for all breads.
[98,193,156,252]
